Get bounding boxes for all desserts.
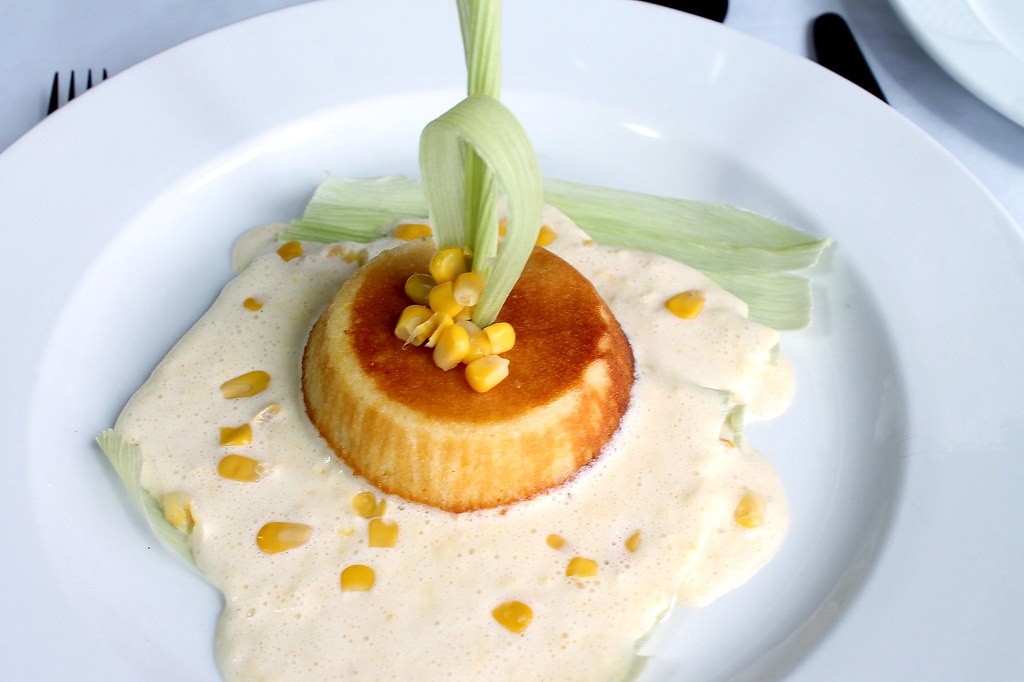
[301,0,638,515]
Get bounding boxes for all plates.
[887,0,1024,127]
[0,0,1024,682]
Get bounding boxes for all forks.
[46,67,108,116]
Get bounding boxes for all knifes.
[812,12,890,107]
